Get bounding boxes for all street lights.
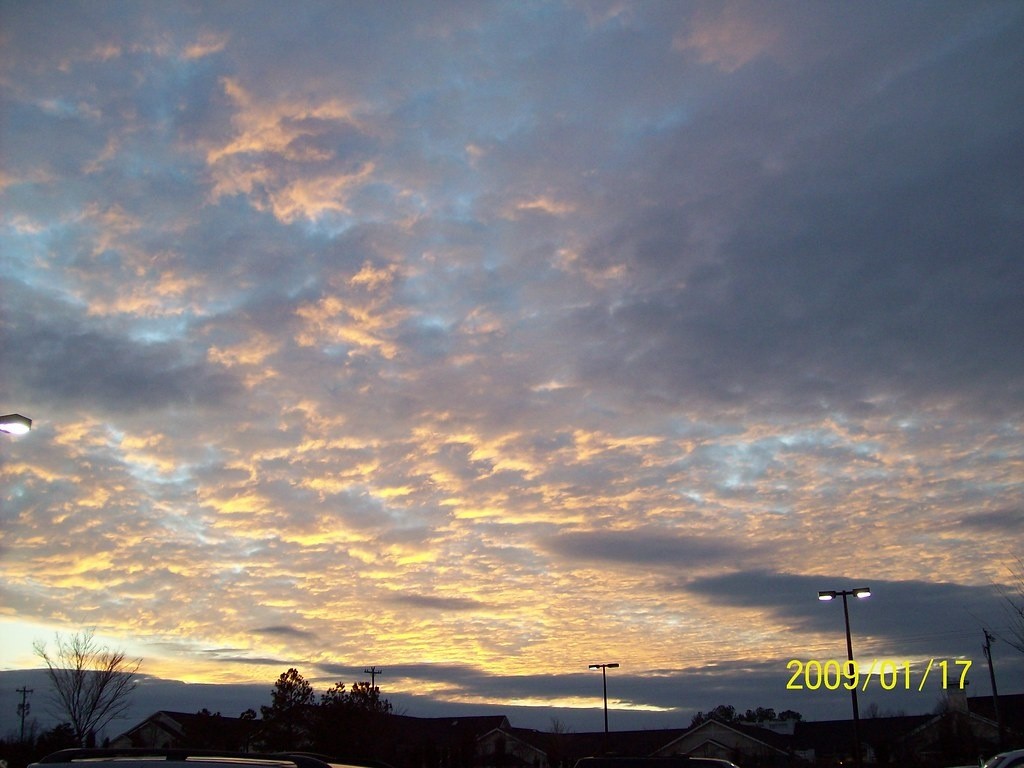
[817,588,870,761]
[591,664,619,734]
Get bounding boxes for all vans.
[573,752,739,768]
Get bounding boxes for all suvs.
[28,748,371,768]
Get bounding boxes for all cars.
[982,749,1024,768]
